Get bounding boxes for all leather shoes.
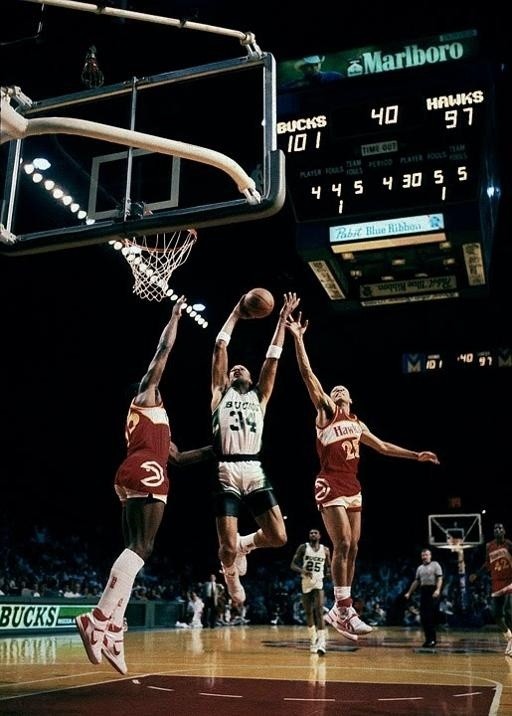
[423,639,436,648]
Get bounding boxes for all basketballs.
[243,287,274,317]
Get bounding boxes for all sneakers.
[326,603,373,640]
[102,620,128,674]
[505,638,512,656]
[310,636,326,653]
[76,611,112,664]
[224,571,247,604]
[235,532,248,576]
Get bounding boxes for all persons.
[130,566,306,628]
[290,528,332,654]
[319,563,422,627]
[284,310,442,644]
[406,550,444,645]
[468,522,512,656]
[1,521,108,598]
[73,293,215,676]
[440,564,497,627]
[210,293,304,606]
